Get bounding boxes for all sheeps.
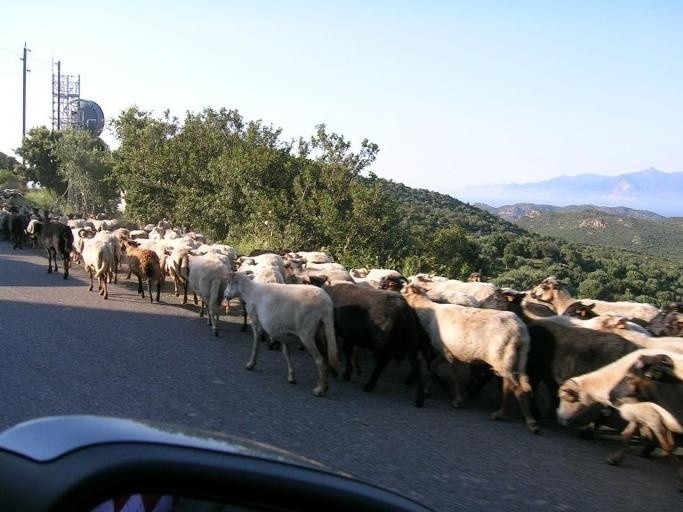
[0,203,683,468]
